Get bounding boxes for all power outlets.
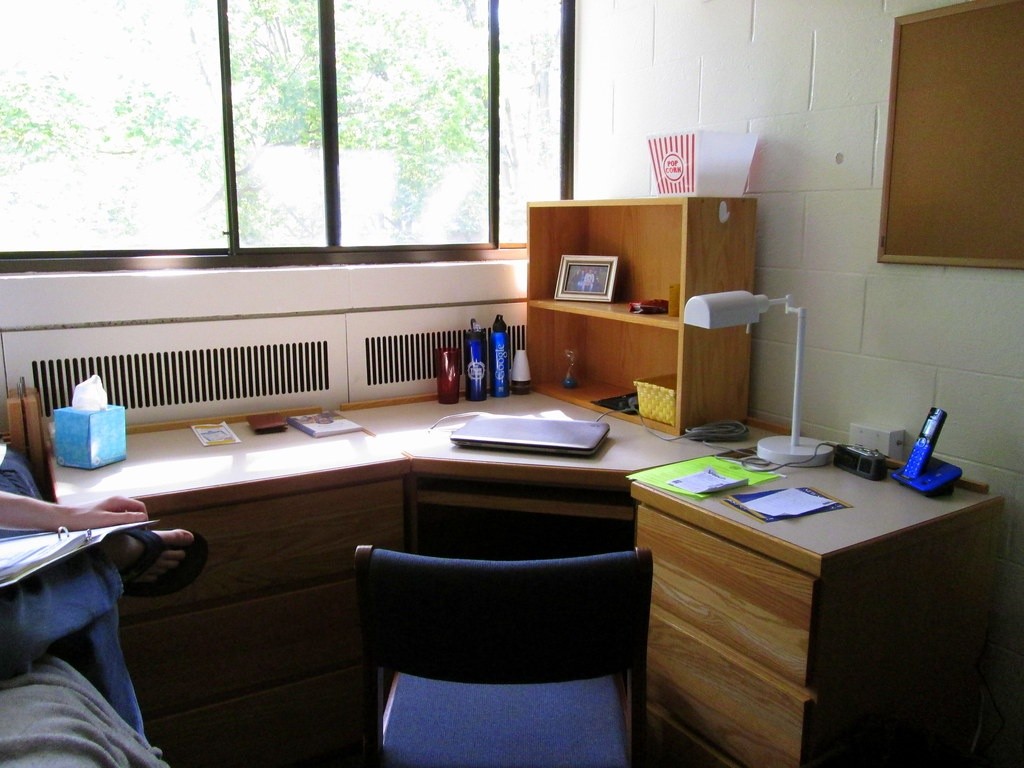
[846,422,905,459]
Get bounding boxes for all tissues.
[52,374,127,471]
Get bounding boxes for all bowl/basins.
[647,130,760,197]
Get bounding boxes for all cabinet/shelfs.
[49,391,1005,768]
[522,193,760,435]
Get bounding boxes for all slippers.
[115,528,210,597]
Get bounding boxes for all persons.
[570,269,602,293]
[0,490,209,739]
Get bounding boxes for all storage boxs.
[644,126,760,198]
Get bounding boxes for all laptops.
[450,414,610,456]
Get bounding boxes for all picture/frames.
[553,254,620,302]
[875,0,1024,271]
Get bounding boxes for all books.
[287,411,363,438]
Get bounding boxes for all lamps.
[684,289,838,468]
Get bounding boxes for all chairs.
[351,541,656,768]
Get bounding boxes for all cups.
[435,348,460,404]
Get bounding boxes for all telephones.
[890,407,963,498]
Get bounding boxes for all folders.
[0,518,161,588]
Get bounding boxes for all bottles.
[490,314,509,398]
[464,317,487,401]
